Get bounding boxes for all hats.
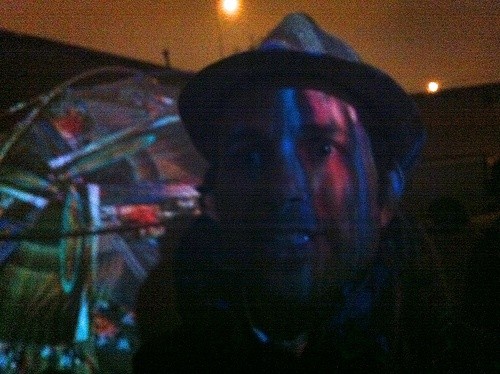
[177,12,425,170]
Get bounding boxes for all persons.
[118,12,480,374]
